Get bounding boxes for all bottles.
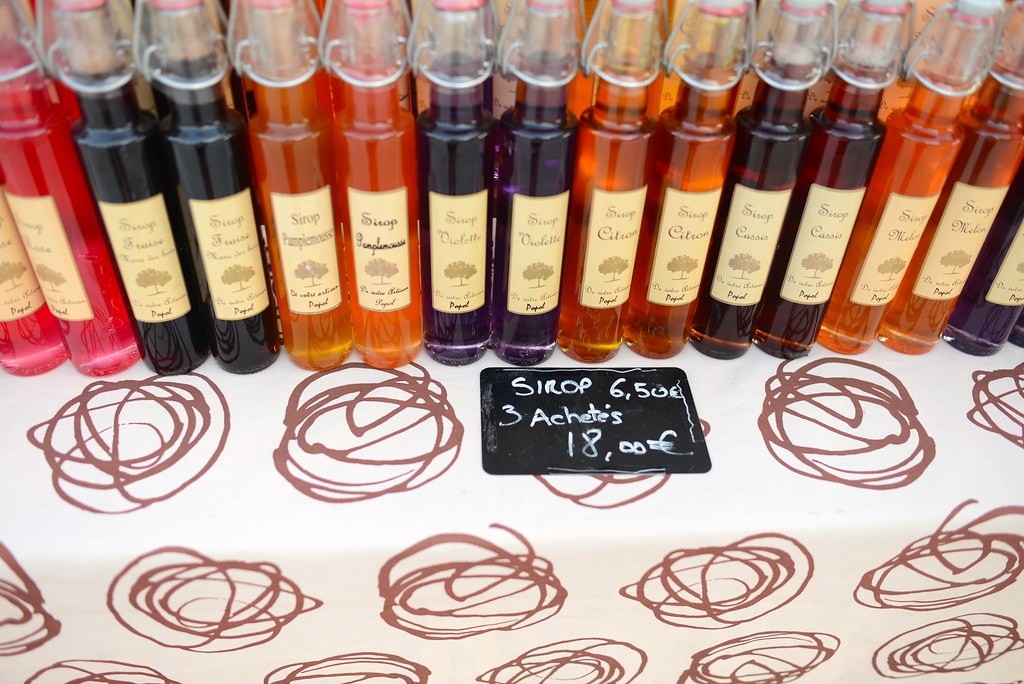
[0,2,1023,376]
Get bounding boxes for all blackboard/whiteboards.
[479,367,713,475]
